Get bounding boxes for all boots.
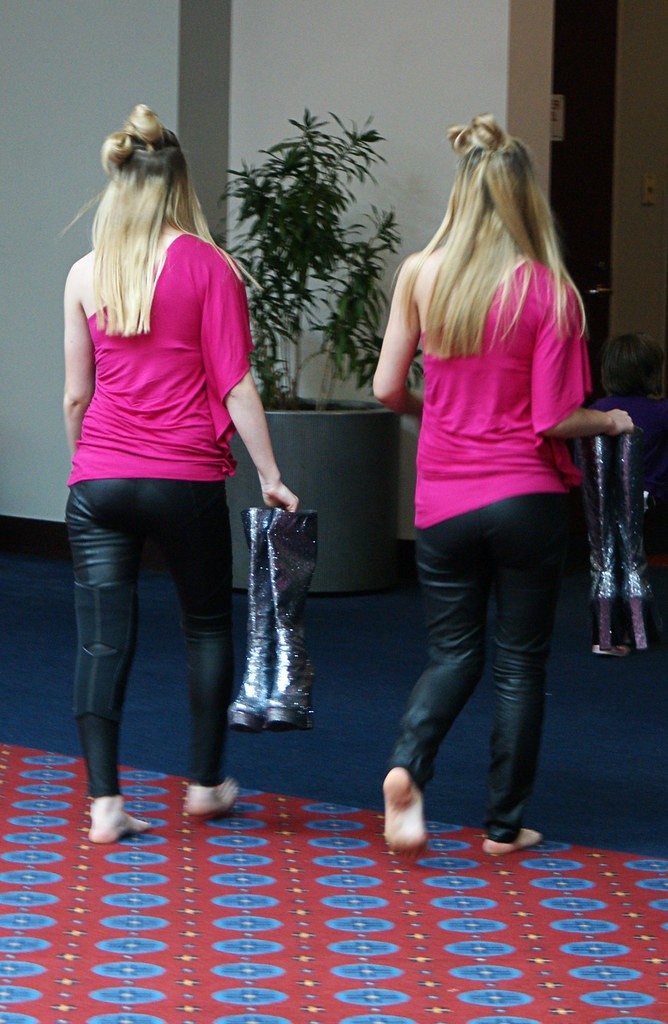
[229,508,319,726]
[580,433,664,653]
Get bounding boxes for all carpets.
[1,744,667,1024]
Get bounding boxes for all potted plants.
[208,108,425,593]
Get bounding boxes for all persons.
[373,112,635,859]
[588,330,668,558]
[64,104,299,844]
